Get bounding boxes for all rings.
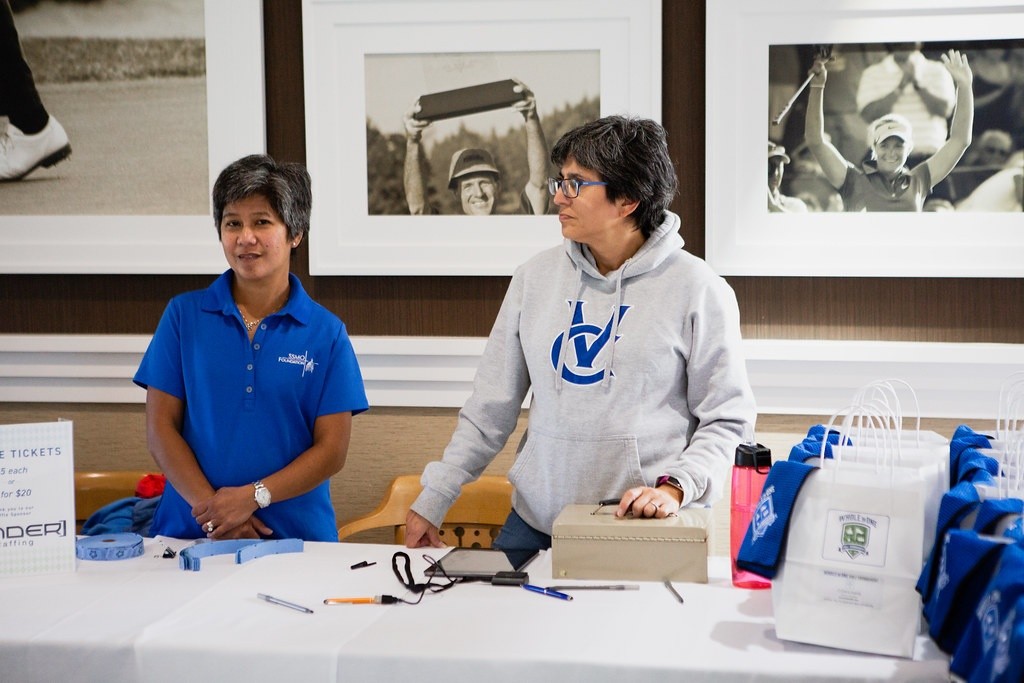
[650,501,659,511]
[206,521,214,532]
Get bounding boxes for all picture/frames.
[0,0,267,275]
[706,0,1023,279]
[301,0,660,278]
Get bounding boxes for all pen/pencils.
[256,592,313,615]
[665,579,684,603]
[323,598,375,603]
[545,585,640,592]
[520,584,573,602]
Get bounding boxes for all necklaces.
[233,298,287,331]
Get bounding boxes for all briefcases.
[550,503,713,584]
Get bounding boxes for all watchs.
[252,480,271,509]
[658,476,684,492]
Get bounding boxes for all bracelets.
[809,83,826,88]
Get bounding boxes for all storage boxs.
[553,502,710,586]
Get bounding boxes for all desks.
[0,534,959,683]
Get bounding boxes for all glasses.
[547,173,607,199]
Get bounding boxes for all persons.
[405,78,550,216]
[405,118,759,573]
[145,153,352,542]
[768,43,1024,212]
[0,2,70,182]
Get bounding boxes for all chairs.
[75,471,147,530]
[335,474,510,552]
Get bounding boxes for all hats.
[448,147,499,186]
[871,115,912,146]
[768,141,790,165]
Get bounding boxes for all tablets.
[423,547,540,578]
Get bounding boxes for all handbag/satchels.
[776,375,1024,683]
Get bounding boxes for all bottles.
[730,441,774,589]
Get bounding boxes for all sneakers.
[2,115,73,180]
